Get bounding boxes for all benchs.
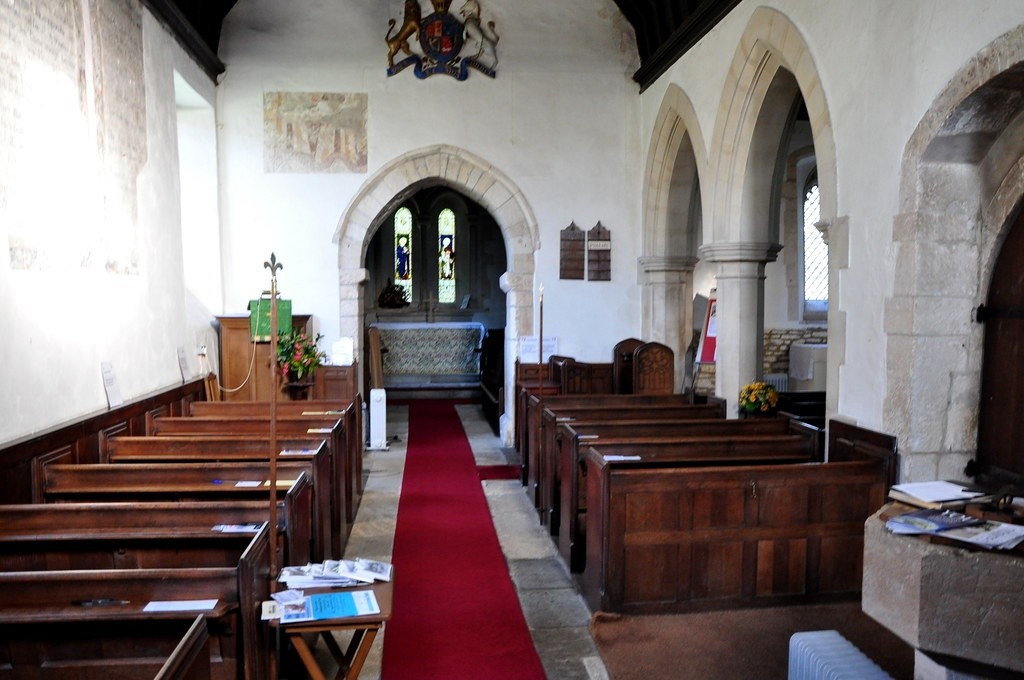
[0,394,373,680]
[525,385,898,617]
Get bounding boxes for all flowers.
[266,328,327,382]
[738,382,778,417]
[378,276,410,309]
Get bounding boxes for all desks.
[269,580,394,680]
[368,322,489,389]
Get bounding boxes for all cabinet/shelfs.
[215,315,312,402]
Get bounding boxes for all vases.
[280,381,315,401]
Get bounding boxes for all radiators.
[788,631,892,680]
[763,373,789,392]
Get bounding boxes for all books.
[264,557,391,622]
[884,480,1024,551]
